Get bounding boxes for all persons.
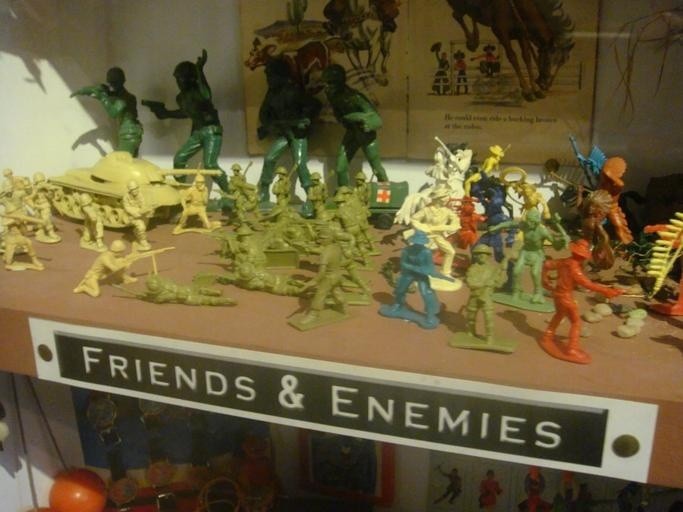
[432,465,463,506]
[518,470,683,512]
[479,470,504,509]
[1,49,682,367]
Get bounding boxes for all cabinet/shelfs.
[0,210,683,512]
